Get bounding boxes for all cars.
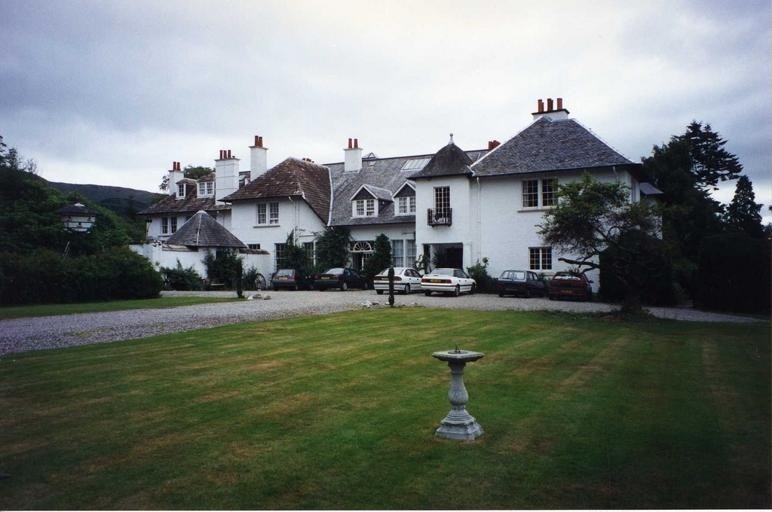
[421,267,477,297]
[272,268,304,291]
[549,271,594,301]
[312,267,371,291]
[373,267,422,294]
[497,269,545,298]
